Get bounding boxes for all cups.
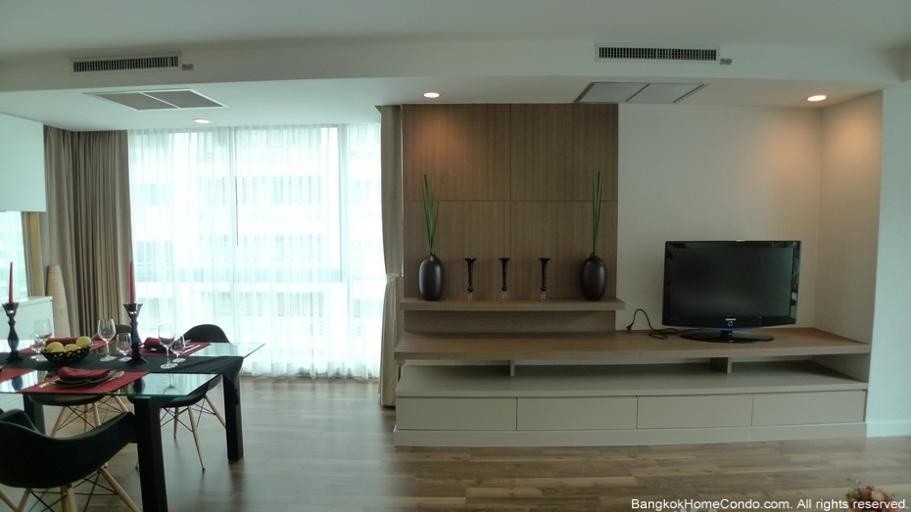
[115,332,133,362]
[34,317,52,361]
[158,324,178,369]
[30,333,45,359]
[169,331,186,363]
[98,319,118,361]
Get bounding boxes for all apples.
[46,341,65,351]
[75,336,91,347]
[66,344,83,351]
[52,348,66,357]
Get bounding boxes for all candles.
[8,261,14,303]
[128,257,135,304]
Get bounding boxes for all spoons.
[94,370,125,390]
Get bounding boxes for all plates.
[56,372,113,387]
[41,345,91,366]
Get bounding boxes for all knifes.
[38,377,59,389]
[182,344,201,355]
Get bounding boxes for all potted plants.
[415,167,445,303]
[578,169,609,300]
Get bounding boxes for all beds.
[0,342,263,512]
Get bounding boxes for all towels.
[43,336,87,345]
[143,335,183,352]
[57,364,109,381]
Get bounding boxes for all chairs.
[50,325,142,435]
[159,324,227,473]
[0,407,135,511]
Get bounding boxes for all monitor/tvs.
[660,239,803,344]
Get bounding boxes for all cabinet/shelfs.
[390,328,871,446]
